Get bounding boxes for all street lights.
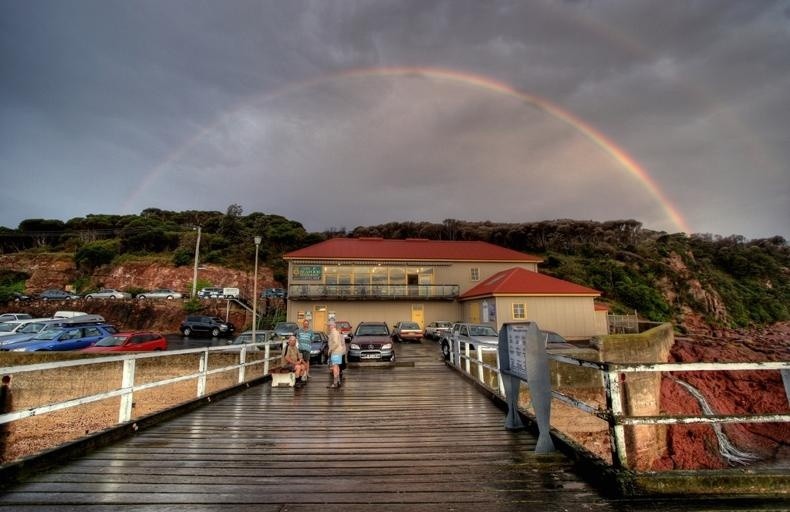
[251,235,263,344]
[192,224,202,298]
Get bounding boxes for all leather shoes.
[296,381,306,388]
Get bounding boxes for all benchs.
[269,366,308,387]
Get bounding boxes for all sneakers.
[330,383,341,388]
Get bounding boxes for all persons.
[282,335,308,388]
[0,376,13,415]
[327,321,344,389]
[329,328,346,378]
[298,319,314,377]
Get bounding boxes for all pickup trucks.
[439,322,499,361]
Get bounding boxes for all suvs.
[346,321,396,362]
[179,316,235,337]
[197,286,235,299]
[271,321,301,340]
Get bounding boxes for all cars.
[296,331,329,365]
[260,287,287,299]
[393,321,424,343]
[335,320,353,342]
[424,321,454,340]
[0,310,168,355]
[227,329,276,352]
[135,288,182,301]
[8,288,132,303]
[508,326,578,350]
